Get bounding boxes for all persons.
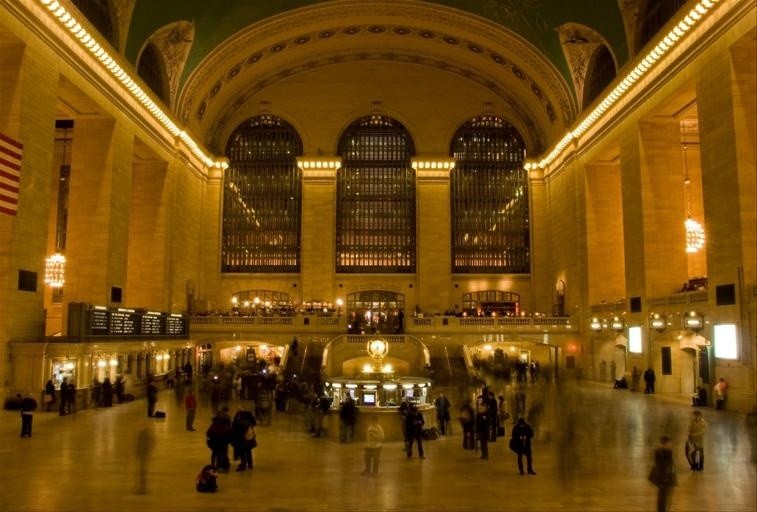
[443,304,506,325]
[644,367,656,394]
[682,281,705,292]
[687,410,709,472]
[631,365,640,392]
[654,435,677,512]
[353,309,362,331]
[42,379,56,413]
[89,353,549,494]
[58,377,68,417]
[397,308,404,331]
[714,378,727,410]
[188,303,342,328]
[67,383,76,414]
[691,385,708,407]
[18,392,37,439]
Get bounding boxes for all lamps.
[684,146,704,254]
[43,128,68,288]
